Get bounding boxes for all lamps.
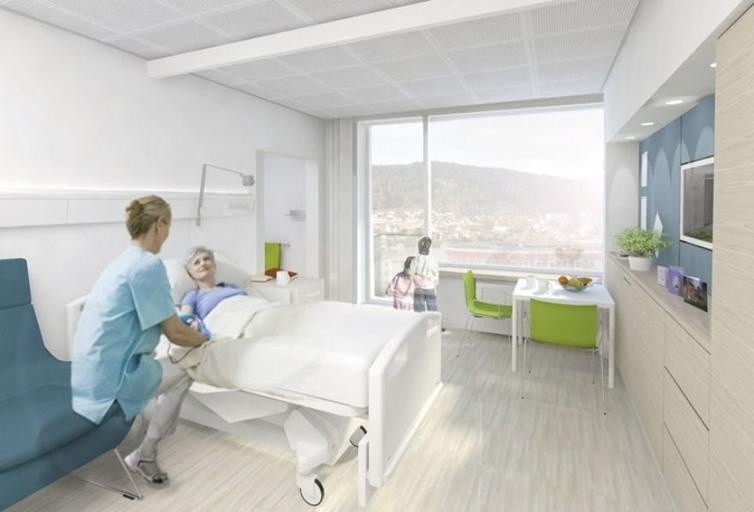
[192,159,256,227]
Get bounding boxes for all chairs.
[458,270,514,355]
[521,297,611,405]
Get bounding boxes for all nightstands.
[247,275,324,302]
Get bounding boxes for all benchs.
[1,256,140,512]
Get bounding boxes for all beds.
[59,255,441,512]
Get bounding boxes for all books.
[263,241,281,271]
[263,267,299,280]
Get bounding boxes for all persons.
[180,244,251,339]
[68,193,205,486]
[385,255,416,310]
[695,285,703,300]
[413,235,446,333]
[685,282,703,305]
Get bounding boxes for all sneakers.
[125,449,168,484]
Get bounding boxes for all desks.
[511,276,615,391]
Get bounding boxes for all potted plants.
[610,226,671,271]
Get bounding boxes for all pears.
[568,278,592,288]
